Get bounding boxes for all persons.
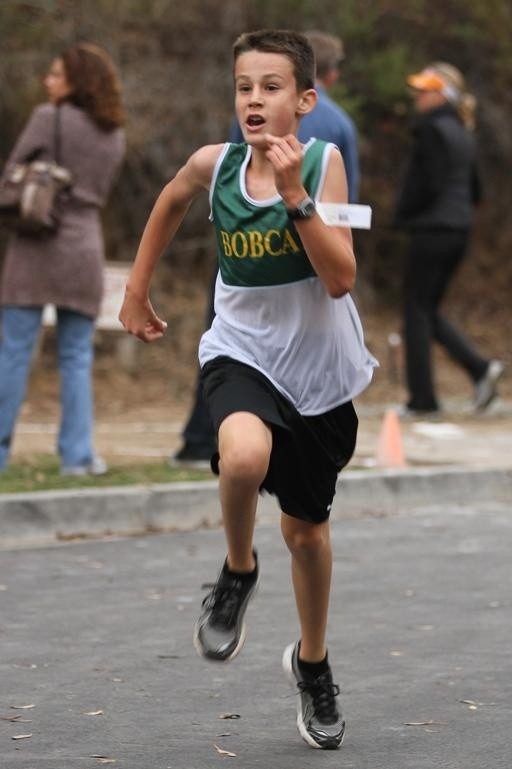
[389,62,503,422]
[176,28,360,468]
[1,40,128,484]
[118,30,381,751]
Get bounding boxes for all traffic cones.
[370,401,409,470]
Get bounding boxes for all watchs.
[283,198,316,221]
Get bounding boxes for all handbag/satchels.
[0,104,75,239]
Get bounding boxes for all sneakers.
[282,637,345,750]
[193,546,261,663]
[472,359,505,413]
[388,398,446,424]
[163,445,217,473]
[60,454,107,479]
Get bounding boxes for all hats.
[405,66,459,111]
